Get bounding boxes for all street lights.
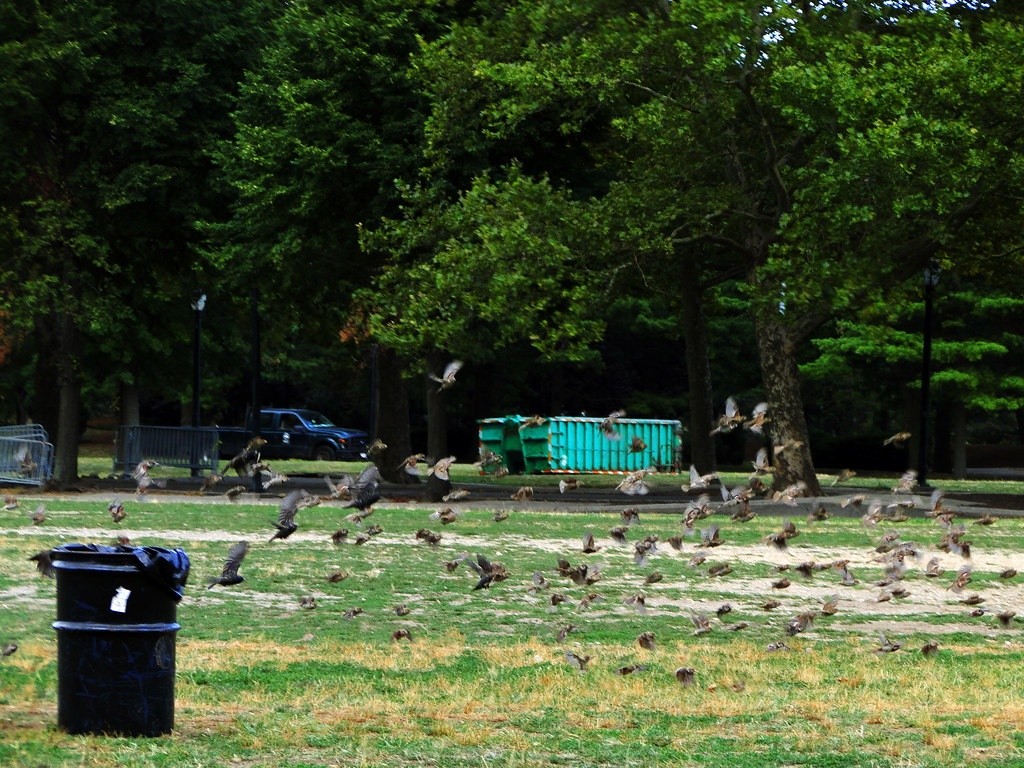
[914,261,942,487]
[190,294,208,476]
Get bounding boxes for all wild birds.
[0,359,1018,686]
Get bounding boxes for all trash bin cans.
[52,544,182,738]
[477,414,682,476]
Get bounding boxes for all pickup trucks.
[179,409,369,462]
